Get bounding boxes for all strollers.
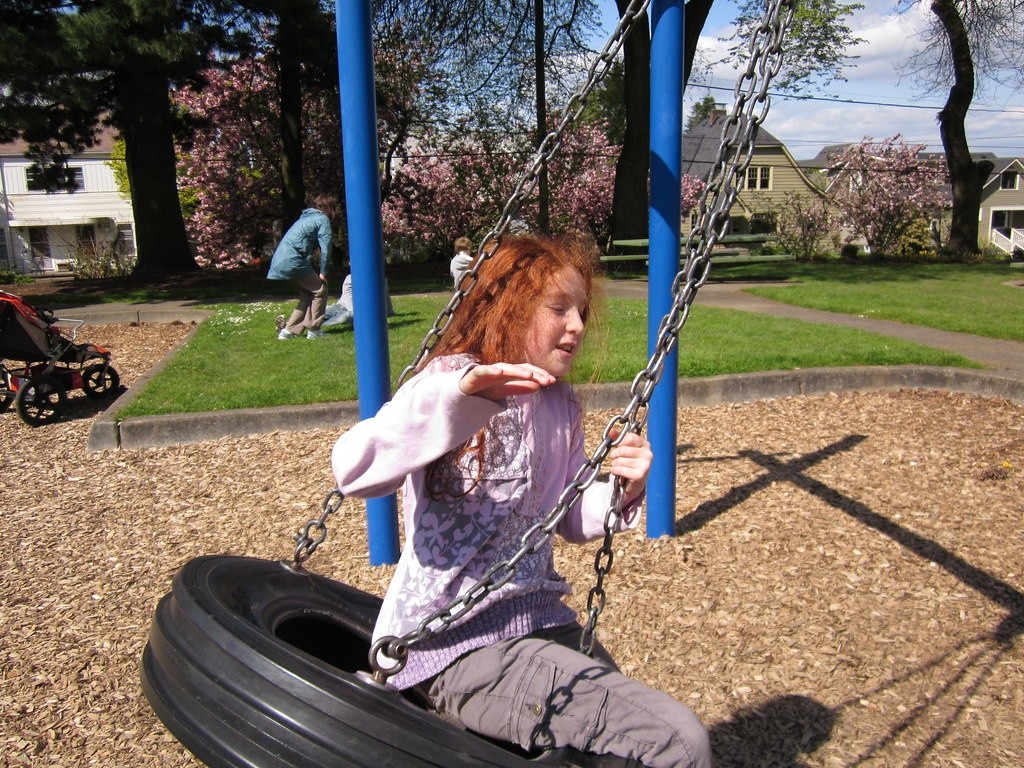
[0,290,120,426]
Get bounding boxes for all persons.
[330,234,713,768]
[449,236,473,286]
[274,273,394,333]
[266,191,338,340]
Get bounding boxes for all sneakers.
[307,329,331,339]
[278,329,295,340]
